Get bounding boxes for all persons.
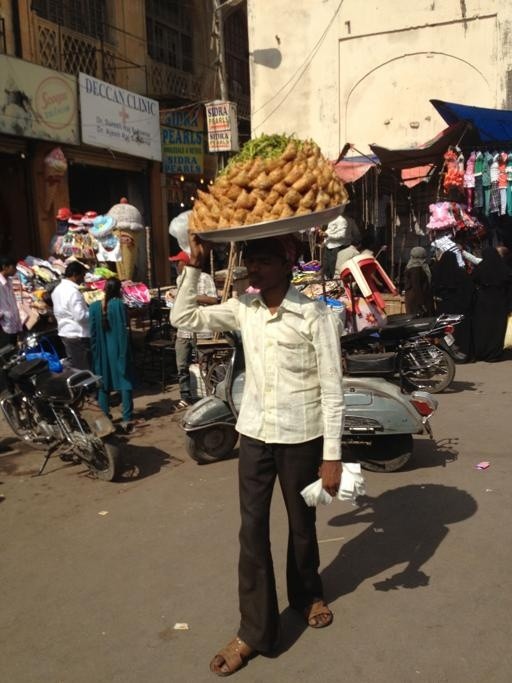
[168,228,351,676]
[444,143,512,217]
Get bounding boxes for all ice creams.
[42,146,68,213]
[106,196,145,281]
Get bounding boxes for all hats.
[55,207,115,237]
[167,250,201,267]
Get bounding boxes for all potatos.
[189,138,349,233]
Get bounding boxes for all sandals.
[167,399,189,413]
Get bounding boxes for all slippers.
[302,596,332,628]
[209,635,258,677]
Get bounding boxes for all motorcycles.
[179,329,437,473]
[340,307,466,393]
[3,328,120,482]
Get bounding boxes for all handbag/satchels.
[23,334,63,373]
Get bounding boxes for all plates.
[191,200,351,244]
